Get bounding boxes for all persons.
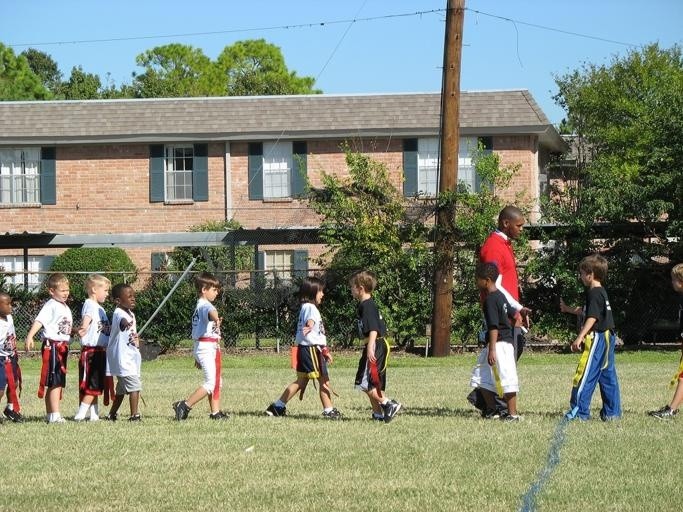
[558,254,622,422]
[649,264,683,420]
[466,206,533,419]
[75,275,114,421]
[348,270,402,423]
[0,293,24,424]
[105,282,144,421]
[23,274,74,423]
[172,271,229,421]
[264,276,343,419]
[470,262,523,424]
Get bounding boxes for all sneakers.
[649,405,680,421]
[172,400,192,420]
[265,403,286,417]
[45,413,142,423]
[210,410,229,420]
[323,408,343,419]
[467,388,520,423]
[3,406,24,423]
[372,399,401,423]
